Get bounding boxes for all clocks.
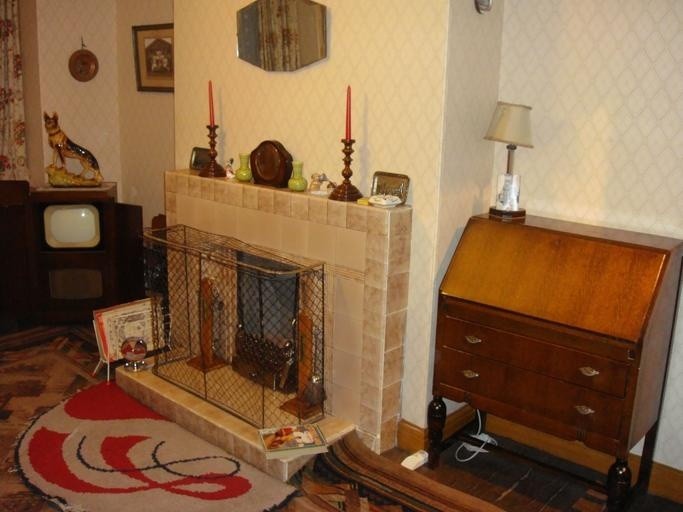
[473,1,493,16]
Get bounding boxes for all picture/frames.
[132,23,174,93]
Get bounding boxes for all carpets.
[10,377,302,511]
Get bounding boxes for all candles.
[346,85,352,142]
[208,80,215,128]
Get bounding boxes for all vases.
[236,153,253,182]
[287,161,308,192]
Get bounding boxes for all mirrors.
[236,0,327,72]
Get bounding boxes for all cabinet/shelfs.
[428,212,683,512]
[28,180,146,331]
[0,181,28,334]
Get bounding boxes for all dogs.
[44,111,103,186]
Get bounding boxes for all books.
[256,422,329,460]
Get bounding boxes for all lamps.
[483,100,534,220]
